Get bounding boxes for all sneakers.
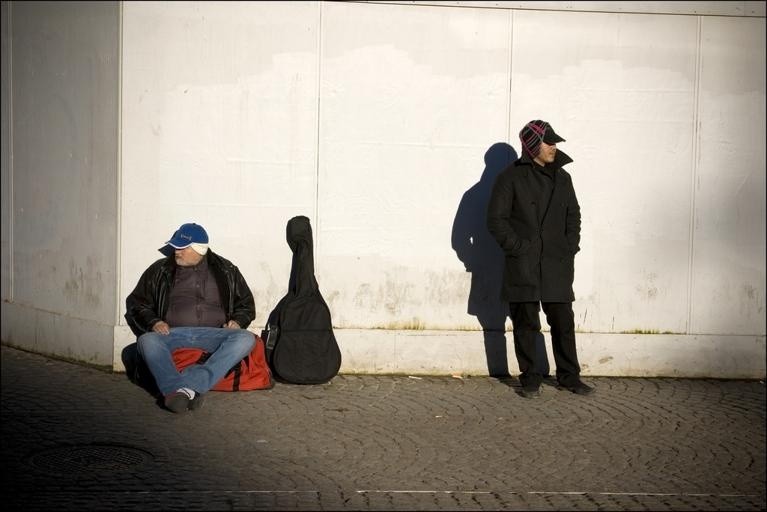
[519,377,545,400]
[163,383,204,414]
[558,379,598,396]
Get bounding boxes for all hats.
[164,222,209,250]
[518,119,565,156]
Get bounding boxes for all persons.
[123,222,258,414]
[486,119,594,399]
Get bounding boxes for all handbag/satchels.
[156,334,272,393]
